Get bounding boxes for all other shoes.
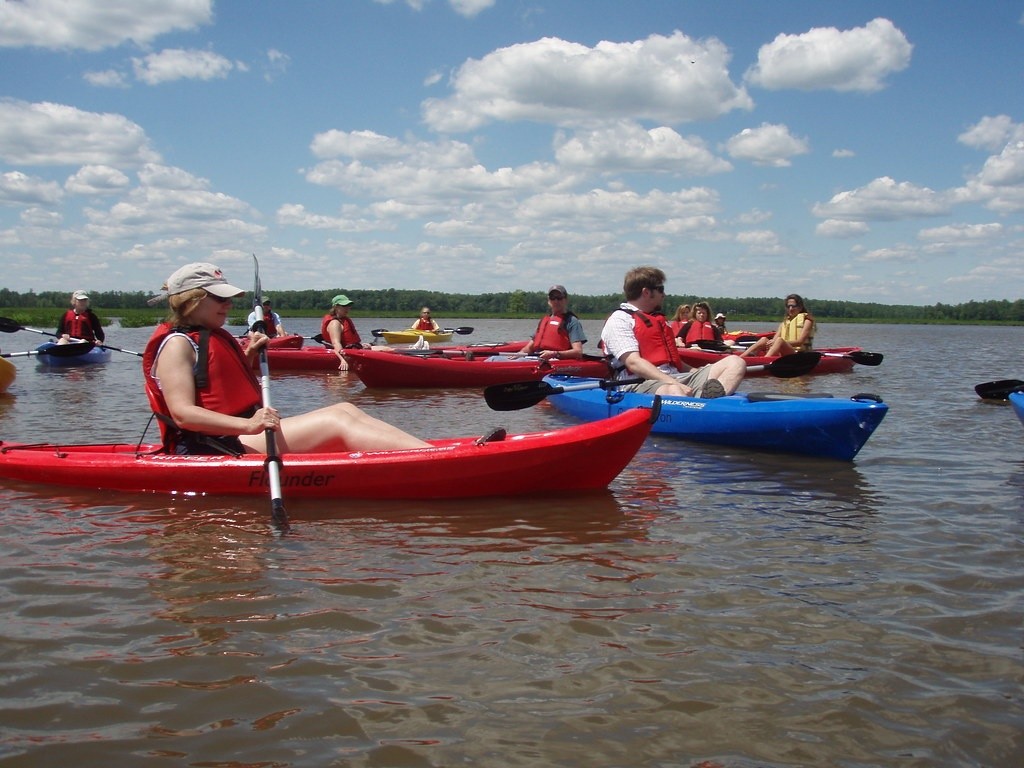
[474,428,507,443]
[700,379,725,401]
[410,335,430,350]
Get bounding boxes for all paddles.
[371,326,475,336]
[233,334,324,344]
[484,350,822,412]
[0,316,144,357]
[975,379,1024,399]
[695,339,883,366]
[252,254,288,520]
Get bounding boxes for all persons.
[142,263,506,458]
[668,302,730,351]
[56,290,105,346]
[412,307,439,335]
[739,294,815,357]
[484,285,587,362]
[322,295,430,370]
[248,296,285,339]
[601,266,746,399]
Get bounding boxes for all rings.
[272,419,274,424]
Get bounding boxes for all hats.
[73,290,89,299]
[547,285,567,295]
[261,296,269,304]
[167,263,245,298]
[714,313,726,319]
[332,295,354,307]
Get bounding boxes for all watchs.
[555,351,561,359]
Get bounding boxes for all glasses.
[786,304,797,308]
[649,286,664,293]
[205,293,225,303]
[548,295,565,300]
[422,312,430,315]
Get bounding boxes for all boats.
[377,331,454,344]
[542,370,889,460]
[0,395,662,497]
[675,330,857,378]
[237,332,344,371]
[338,342,616,391]
[35,341,112,364]
